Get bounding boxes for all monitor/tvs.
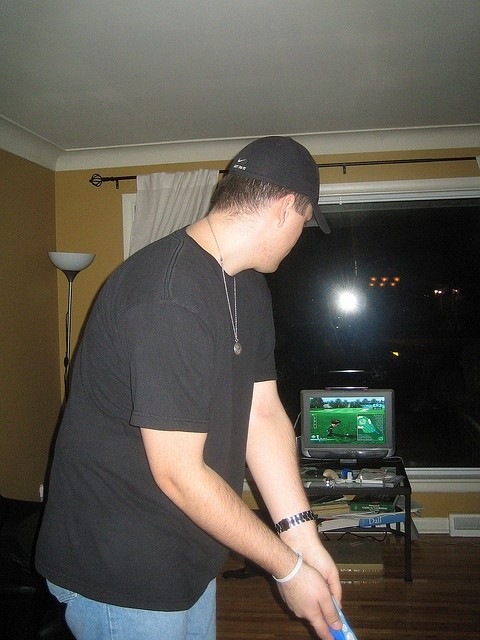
[300,389,395,458]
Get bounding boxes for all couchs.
[0,492,73,640]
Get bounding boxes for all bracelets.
[275,509,316,535]
[272,551,303,583]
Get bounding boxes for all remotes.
[385,475,405,488]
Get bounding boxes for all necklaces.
[206,217,243,356]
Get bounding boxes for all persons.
[34,136,343,639]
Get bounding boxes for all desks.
[299,458,413,583]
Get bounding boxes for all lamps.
[48,250,95,372]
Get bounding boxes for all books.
[308,497,350,517]
[317,513,406,532]
[349,495,399,512]
[355,468,387,484]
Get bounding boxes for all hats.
[228,136,330,233]
